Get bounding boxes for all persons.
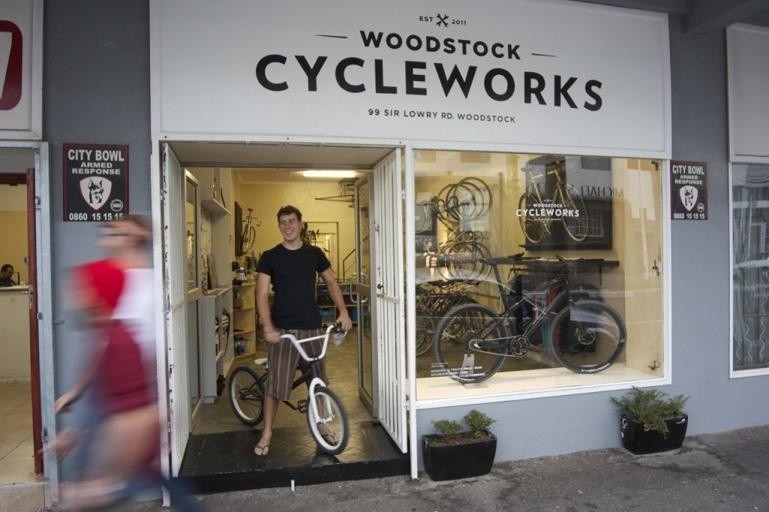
[52,211,165,511]
[253,204,353,457]
[34,258,163,510]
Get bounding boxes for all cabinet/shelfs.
[198,286,234,405]
[234,282,257,361]
[318,283,357,325]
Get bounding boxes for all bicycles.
[240,207,256,255]
[302,229,329,256]
[415,160,492,350]
[433,251,627,384]
[518,158,588,245]
[227,322,349,455]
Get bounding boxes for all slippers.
[321,431,338,445]
[253,436,271,456]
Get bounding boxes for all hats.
[77,260,125,309]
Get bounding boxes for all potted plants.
[423,409,496,481]
[610,386,691,454]
